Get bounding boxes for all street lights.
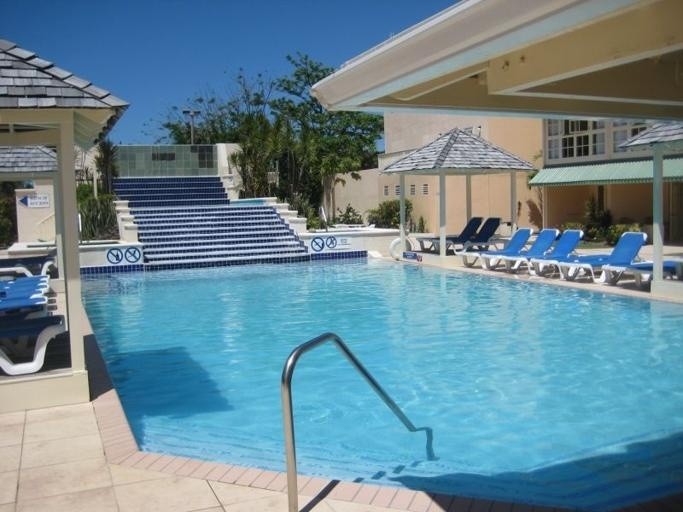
[181,110,199,144]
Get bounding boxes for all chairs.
[0,258,65,376]
[417,216,683,289]
